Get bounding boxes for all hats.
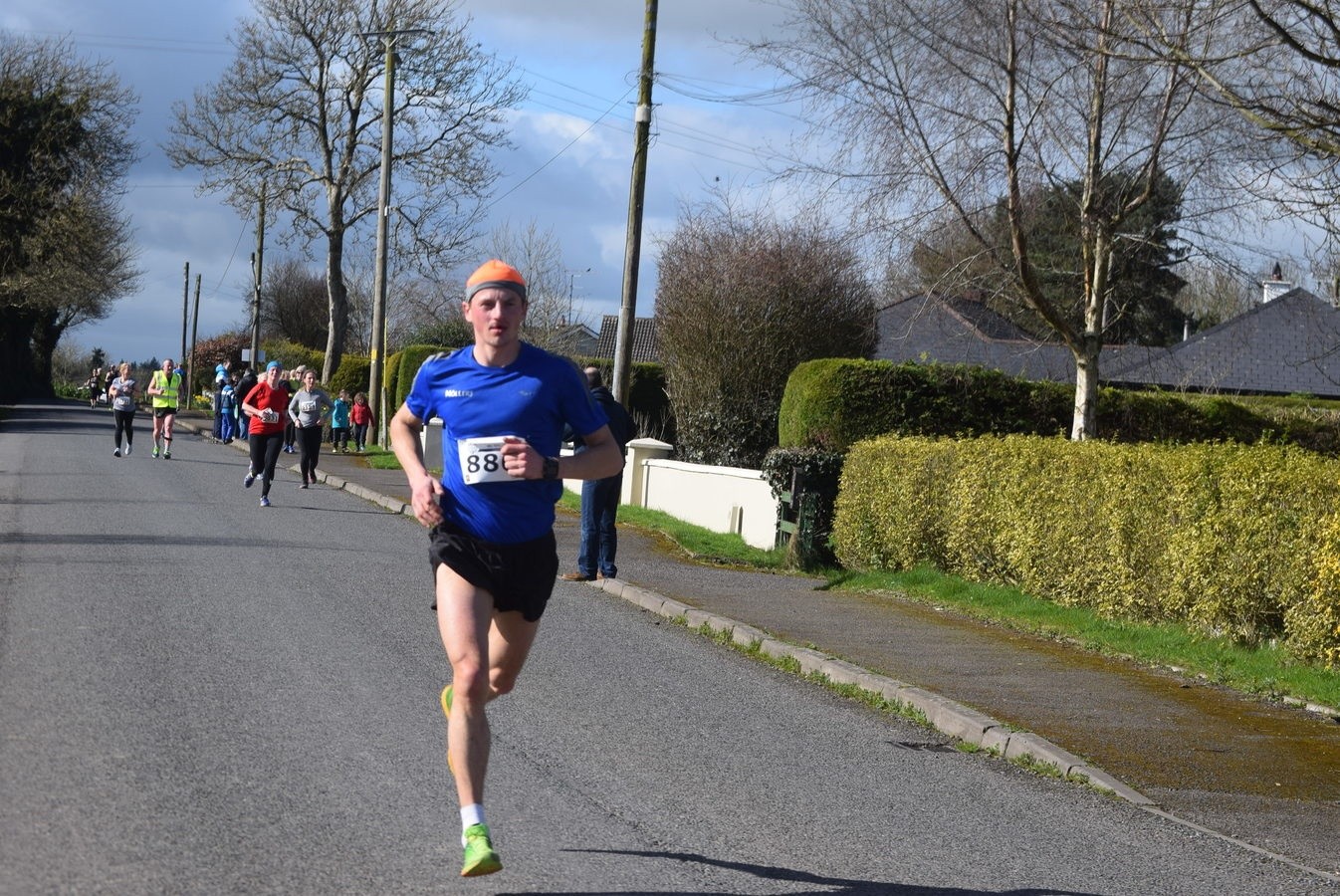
[466,258,526,304]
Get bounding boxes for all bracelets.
[130,389,134,394]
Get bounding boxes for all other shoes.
[163,451,171,459]
[342,448,349,453]
[113,448,121,457]
[556,572,586,581]
[332,446,337,452]
[249,462,263,480]
[244,471,254,488]
[310,475,316,484]
[152,447,159,458]
[283,445,288,452]
[224,438,233,443]
[596,572,604,579]
[360,446,365,451]
[355,448,359,452]
[125,442,132,455]
[260,495,269,506]
[288,445,294,454]
[300,483,308,489]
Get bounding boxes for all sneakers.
[461,822,503,877]
[440,684,455,774]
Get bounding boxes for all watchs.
[543,455,559,481]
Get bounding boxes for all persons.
[391,259,623,878]
[86,365,121,409]
[173,362,185,411]
[109,363,142,457]
[288,368,336,489]
[242,361,289,506]
[212,358,376,454]
[147,359,184,460]
[563,366,638,582]
